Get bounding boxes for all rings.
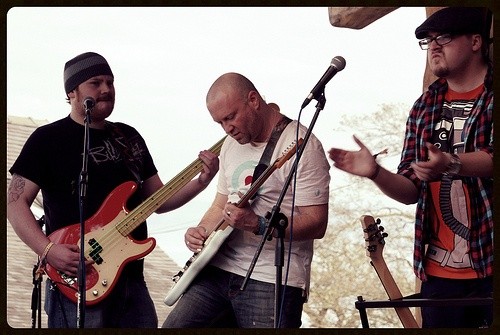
[186,242,190,245]
[227,212,230,216]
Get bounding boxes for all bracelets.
[368,149,389,179]
[41,242,54,257]
[254,215,265,235]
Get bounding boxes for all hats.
[415,6,492,39]
[64,52,114,94]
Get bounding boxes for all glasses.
[419,33,472,50]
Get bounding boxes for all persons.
[160,73,332,328]
[7,52,220,329]
[328,5,494,329]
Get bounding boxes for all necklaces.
[250,107,276,149]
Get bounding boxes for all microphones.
[83,96,95,116]
[301,56,346,110]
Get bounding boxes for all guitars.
[360,214,419,328]
[43,133,228,306]
[164,136,305,308]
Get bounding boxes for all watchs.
[443,151,463,178]
